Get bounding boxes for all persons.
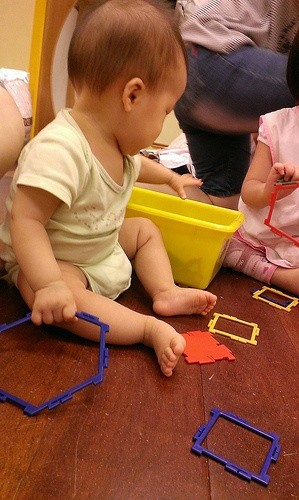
[133,0,299,210]
[222,28,299,299]
[0,1,217,378]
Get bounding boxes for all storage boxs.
[124,186,244,290]
